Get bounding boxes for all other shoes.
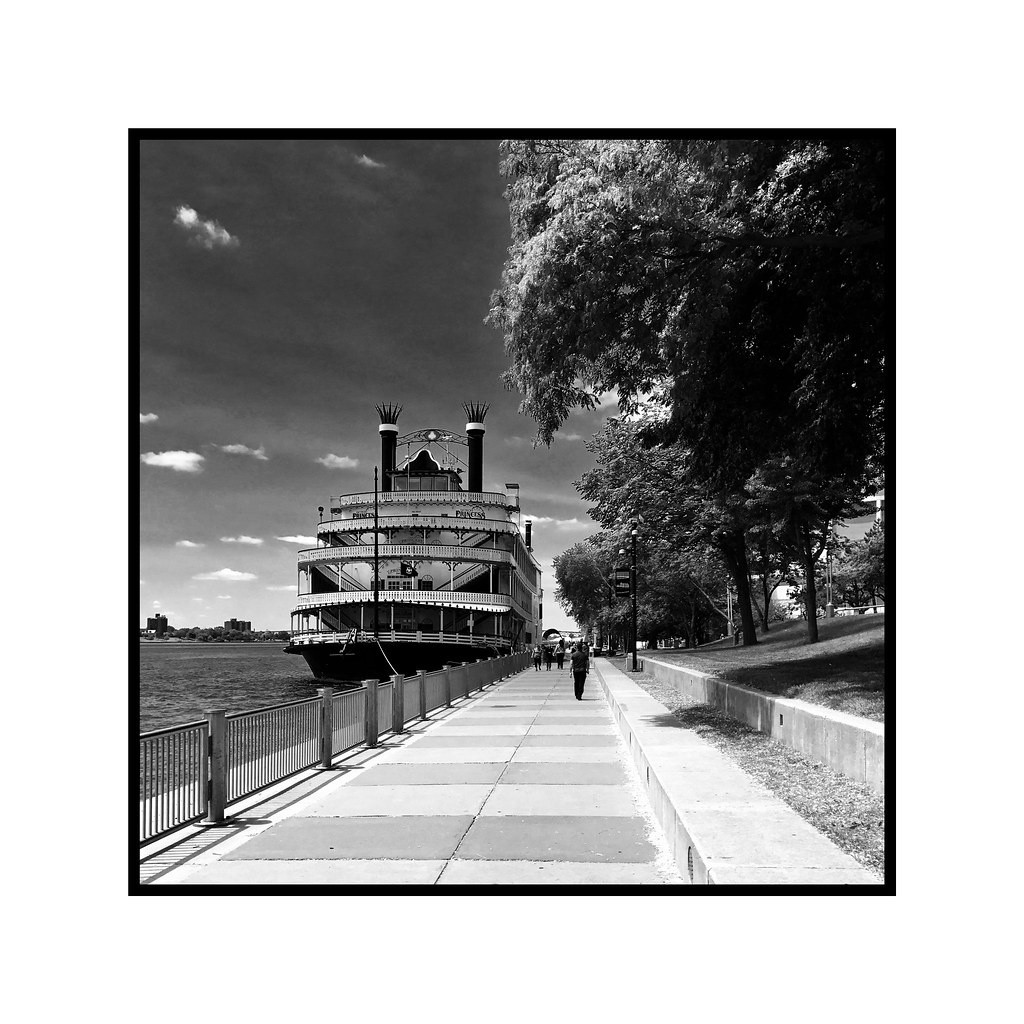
[557,668,559,670]
[578,699,582,700]
[560,668,563,671]
[549,670,551,671]
[536,670,537,671]
[546,670,548,671]
[576,697,578,699]
[539,669,541,670]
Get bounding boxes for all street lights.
[619,530,637,672]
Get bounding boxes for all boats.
[281,398,546,686]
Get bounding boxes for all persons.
[570,642,590,700]
[531,645,554,671]
[734,625,740,645]
[568,641,571,650]
[553,640,565,670]
